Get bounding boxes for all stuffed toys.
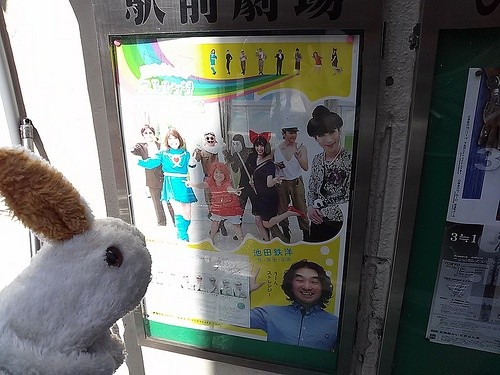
[0,145,151,375]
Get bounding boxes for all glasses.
[143,131,152,134]
[206,137,215,141]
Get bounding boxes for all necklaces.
[323,146,342,165]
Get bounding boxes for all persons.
[331,48,344,76]
[240,50,247,75]
[312,52,323,74]
[209,48,217,75]
[307,105,352,243]
[294,47,302,75]
[274,49,284,75]
[226,50,233,75]
[248,259,339,352]
[256,47,267,75]
[130,121,311,245]
[156,272,247,298]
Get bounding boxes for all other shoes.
[288,206,306,218]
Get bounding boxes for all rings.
[312,219,315,220]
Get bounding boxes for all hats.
[281,118,299,132]
[307,105,343,137]
[232,134,245,145]
